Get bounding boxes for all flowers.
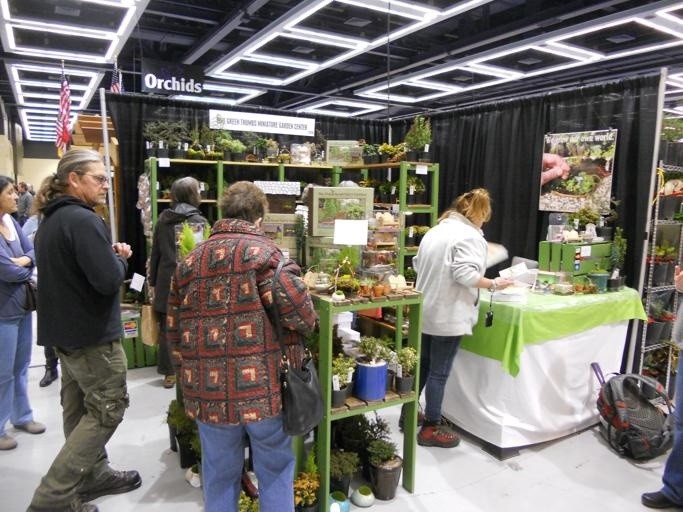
[393,142,408,158]
[378,140,396,155]
[293,473,320,508]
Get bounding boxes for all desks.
[419,286,649,460]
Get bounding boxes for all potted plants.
[174,406,201,468]
[407,183,416,205]
[219,138,248,162]
[190,126,202,151]
[660,118,683,168]
[351,334,391,403]
[238,491,251,512]
[362,437,404,502]
[299,444,318,476]
[184,426,202,488]
[337,409,392,468]
[332,290,345,301]
[415,178,427,204]
[403,269,417,286]
[647,239,679,288]
[278,153,291,164]
[252,138,267,159]
[156,120,172,158]
[642,337,683,401]
[587,269,610,294]
[331,351,357,407]
[349,485,376,507]
[392,346,420,396]
[608,226,627,293]
[248,498,260,512]
[165,400,184,453]
[330,448,360,497]
[168,119,189,159]
[358,284,371,297]
[205,151,215,161]
[187,148,205,160]
[362,143,377,166]
[403,225,416,246]
[404,113,434,162]
[416,225,431,246]
[213,150,223,161]
[373,286,383,297]
[325,490,351,512]
[141,121,158,159]
[404,142,418,161]
[568,205,602,236]
[638,296,676,345]
[264,138,279,158]
[214,129,233,160]
[378,179,391,203]
[389,180,399,204]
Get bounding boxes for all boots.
[39,362,57,387]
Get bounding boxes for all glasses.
[72,168,110,186]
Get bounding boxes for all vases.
[301,502,321,512]
[382,154,390,162]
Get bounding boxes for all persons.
[0,175,47,451]
[17,182,32,227]
[398,188,516,448]
[21,170,61,386]
[165,180,318,512]
[25,148,143,512]
[149,176,209,388]
[541,152,572,188]
[642,265,683,509]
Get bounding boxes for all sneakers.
[16,420,46,435]
[417,421,461,448]
[399,411,427,434]
[0,435,17,450]
[163,372,177,388]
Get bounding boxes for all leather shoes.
[640,490,679,510]
[82,469,142,503]
[73,505,98,512]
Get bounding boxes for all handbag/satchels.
[25,277,40,310]
[271,259,325,436]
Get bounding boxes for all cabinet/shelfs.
[625,67,683,402]
[175,280,424,512]
[143,158,440,284]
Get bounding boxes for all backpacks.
[596,370,675,465]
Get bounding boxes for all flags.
[54,75,72,159]
[110,70,122,94]
